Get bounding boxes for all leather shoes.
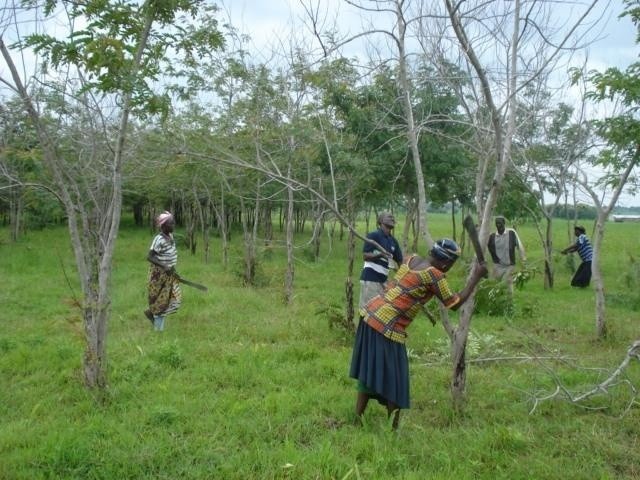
[144,308,154,325]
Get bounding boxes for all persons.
[144,211,182,331]
[561,224,593,287]
[359,212,402,307]
[487,216,527,302]
[348,239,488,431]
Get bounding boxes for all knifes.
[462,215,491,280]
[174,270,209,294]
